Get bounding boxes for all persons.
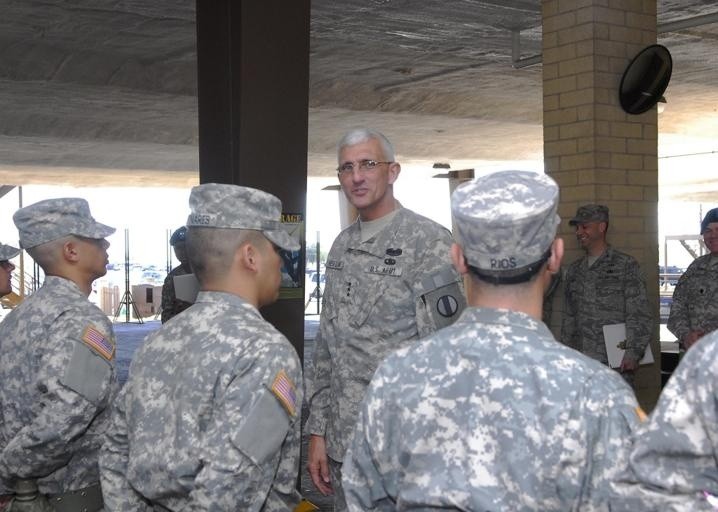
[97,183,305,512]
[560,204,653,385]
[305,125,471,512]
[1,197,119,512]
[162,222,201,324]
[629,328,718,512]
[339,168,649,510]
[0,241,22,299]
[667,205,718,357]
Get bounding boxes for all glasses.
[336,161,394,175]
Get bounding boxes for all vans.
[309,272,327,298]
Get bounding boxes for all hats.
[13,196,117,249]
[449,168,566,273]
[567,203,610,225]
[184,182,302,254]
[0,243,23,263]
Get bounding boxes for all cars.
[107,262,165,283]
[660,266,684,284]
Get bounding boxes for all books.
[601,323,655,369]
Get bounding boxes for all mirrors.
[616,43,674,115]
